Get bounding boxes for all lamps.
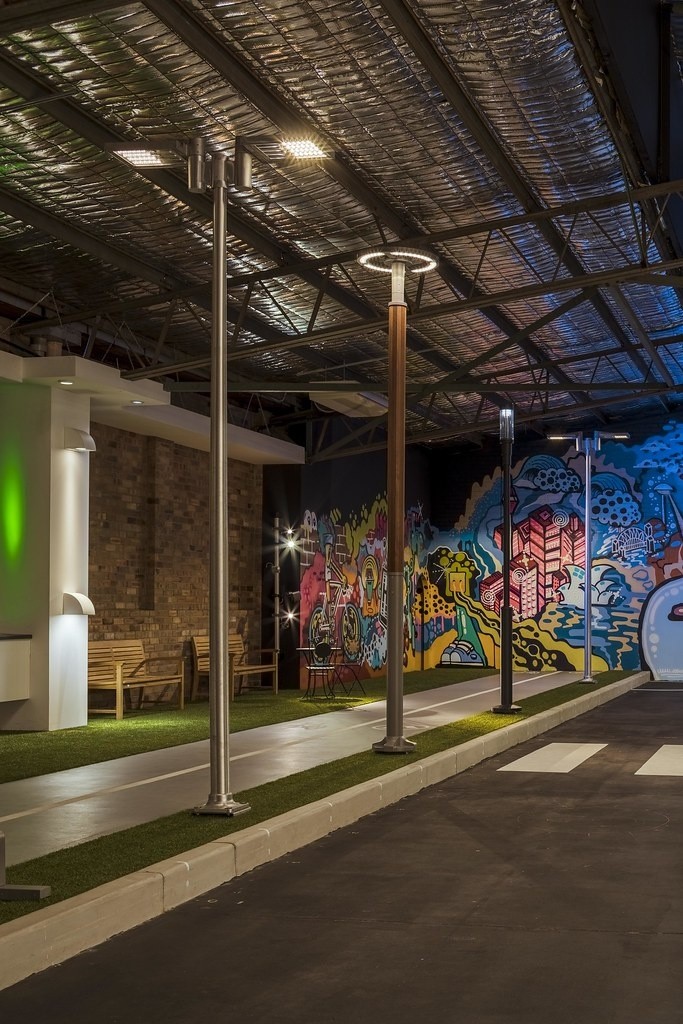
[63,426,96,452]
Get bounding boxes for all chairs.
[327,634,366,697]
[308,634,348,697]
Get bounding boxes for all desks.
[295,647,342,700]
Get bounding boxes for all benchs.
[188,634,280,702]
[88,639,184,720]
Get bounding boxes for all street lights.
[491,400,523,716]
[355,244,439,756]
[547,429,632,684]
[106,131,336,818]
